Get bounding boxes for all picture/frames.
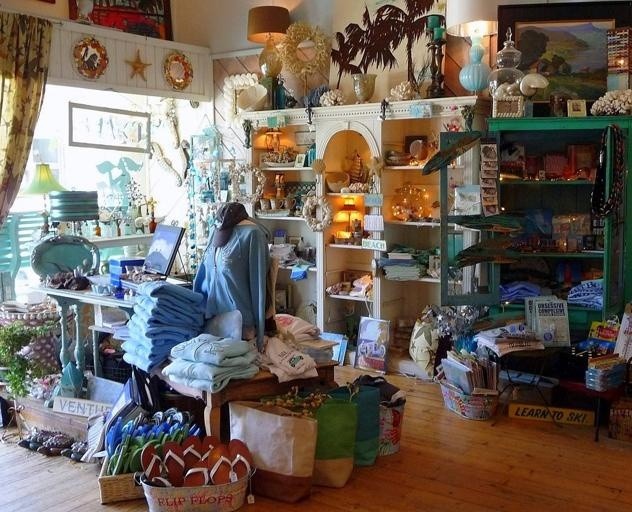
[496,3,632,113]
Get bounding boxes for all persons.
[192,202,270,352]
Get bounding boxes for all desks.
[39,277,345,449]
[86,229,163,250]
[461,330,631,443]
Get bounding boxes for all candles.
[427,16,445,42]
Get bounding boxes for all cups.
[305,247,315,261]
[91,284,116,295]
[259,198,296,211]
[539,319,555,346]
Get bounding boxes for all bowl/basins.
[326,172,350,192]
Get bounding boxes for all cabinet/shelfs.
[241,94,631,380]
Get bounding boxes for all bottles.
[296,237,305,259]
[565,217,578,254]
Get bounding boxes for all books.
[440,296,571,397]
[142,223,187,276]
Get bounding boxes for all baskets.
[98,454,146,504]
[133,466,256,512]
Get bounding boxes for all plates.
[385,149,411,166]
[409,140,428,159]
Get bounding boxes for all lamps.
[24,162,101,242]
[444,0,501,96]
[248,6,292,78]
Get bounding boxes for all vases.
[351,74,377,102]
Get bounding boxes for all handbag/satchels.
[313,395,358,489]
[329,384,381,467]
[353,374,407,457]
[229,400,318,503]
